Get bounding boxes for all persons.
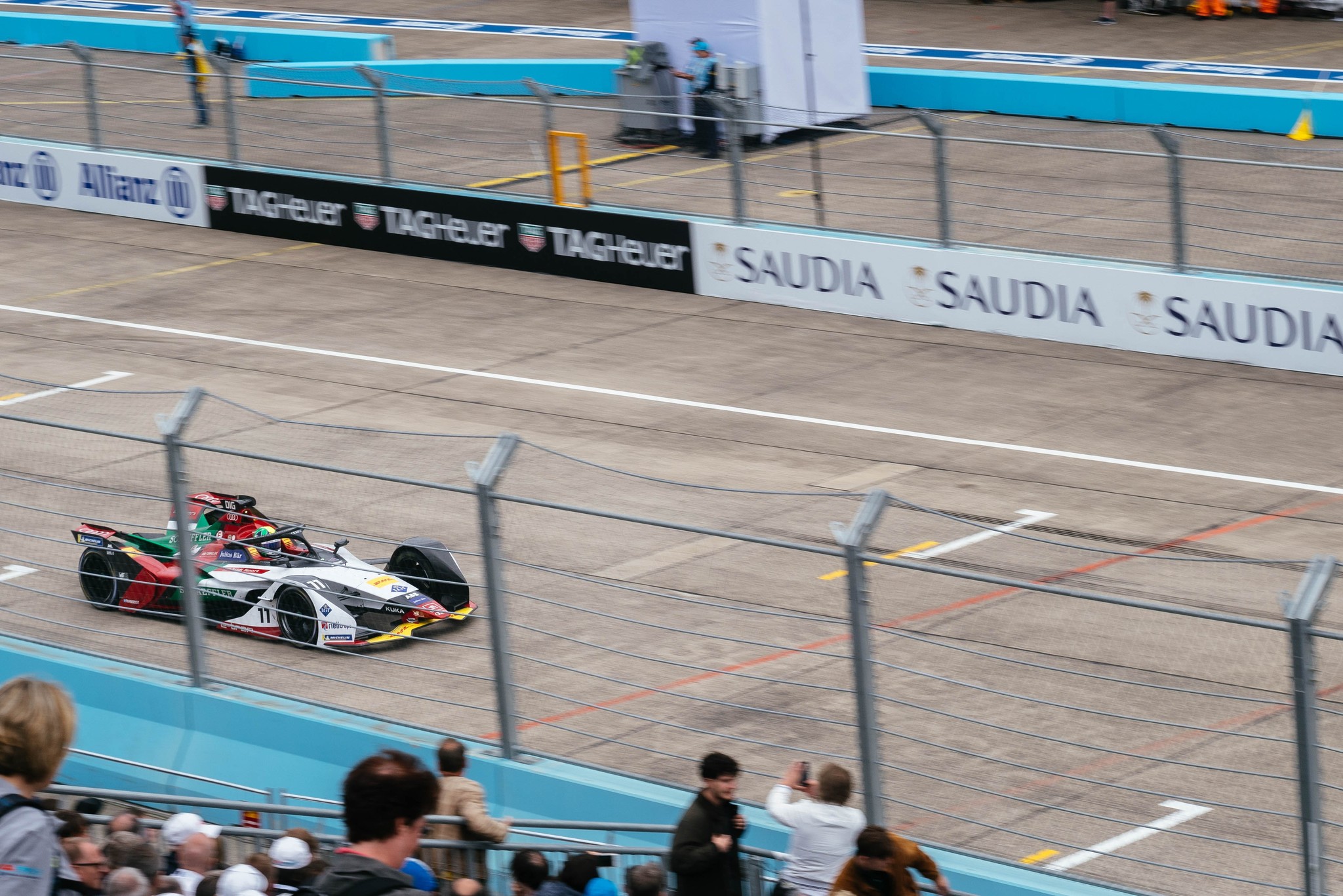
[763,757,867,896]
[177,29,214,129]
[43,796,331,896]
[288,748,493,896]
[420,737,514,885]
[1090,1,1343,26]
[171,1,201,67]
[448,847,675,895]
[247,526,307,564]
[668,41,722,159]
[669,750,748,896]
[828,825,950,896]
[0,675,81,896]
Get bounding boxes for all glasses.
[74,862,110,870]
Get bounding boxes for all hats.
[216,863,269,896]
[689,42,708,51]
[560,859,600,892]
[270,836,313,869]
[162,813,224,846]
[587,878,619,896]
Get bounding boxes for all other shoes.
[702,153,719,158]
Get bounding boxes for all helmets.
[254,526,282,558]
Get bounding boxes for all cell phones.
[801,762,809,787]
